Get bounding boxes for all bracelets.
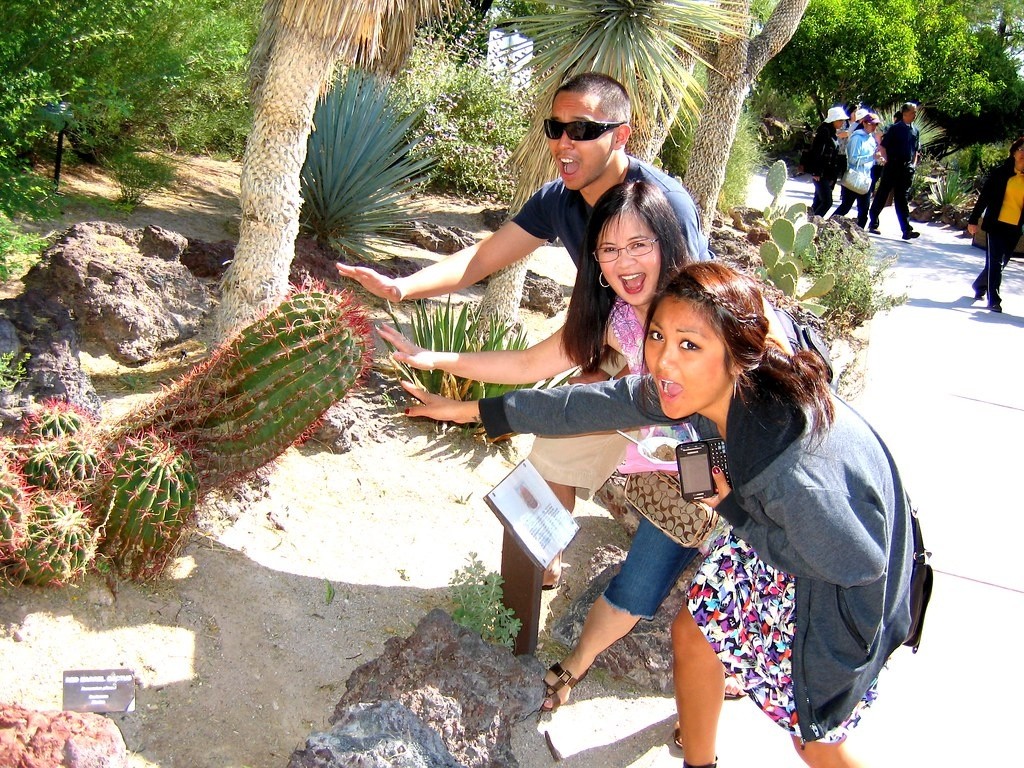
[473,399,482,422]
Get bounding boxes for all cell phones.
[675,437,732,501]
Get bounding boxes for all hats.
[855,109,869,122]
[863,113,882,125]
[823,106,849,124]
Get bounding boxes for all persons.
[336,72,919,768]
[968,136,1024,312]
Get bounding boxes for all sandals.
[541,661,589,712]
[674,721,684,748]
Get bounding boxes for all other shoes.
[988,302,1002,313]
[972,281,985,300]
[902,231,920,240]
[869,228,880,234]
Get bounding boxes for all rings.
[401,339,406,342]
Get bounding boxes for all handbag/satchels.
[899,562,935,650]
[622,468,718,549]
[841,158,873,195]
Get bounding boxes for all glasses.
[544,117,626,142]
[593,237,658,263]
[1017,148,1024,152]
